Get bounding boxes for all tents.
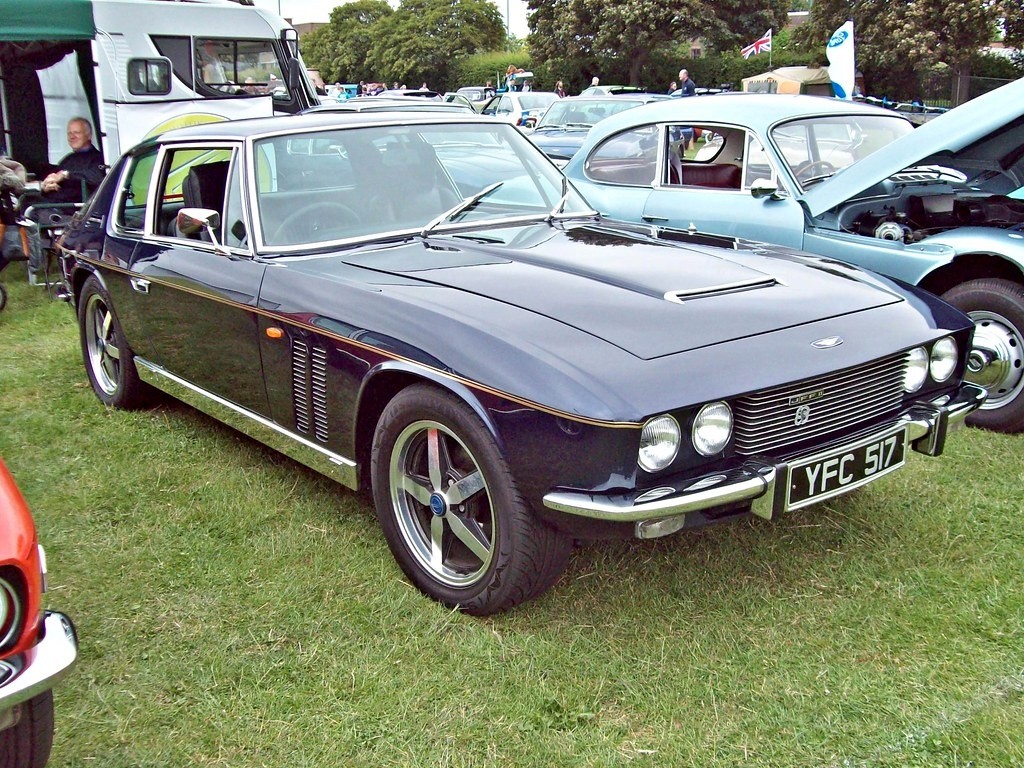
[741,63,836,99]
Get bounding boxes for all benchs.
[590,164,740,188]
[125,187,355,236]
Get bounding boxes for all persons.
[668,69,695,97]
[485,81,495,100]
[419,82,429,91]
[265,74,277,92]
[311,79,406,103]
[17,118,106,222]
[588,77,599,88]
[510,78,533,92]
[554,81,566,98]
[244,77,257,92]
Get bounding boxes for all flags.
[741,28,772,60]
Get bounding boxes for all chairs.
[378,141,460,227]
[18,180,88,286]
[564,112,587,124]
[168,161,230,243]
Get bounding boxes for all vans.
[0,3,323,257]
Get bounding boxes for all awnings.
[0,0,128,159]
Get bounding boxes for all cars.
[52,111,987,617]
[2,455,80,768]
[347,76,1024,430]
